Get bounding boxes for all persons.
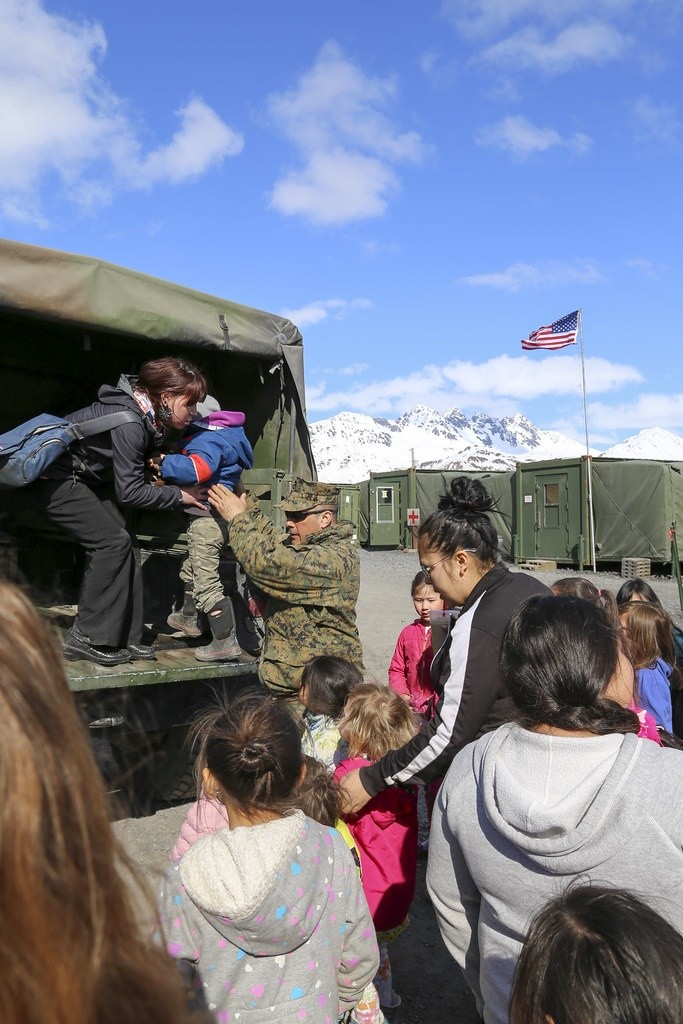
[336,474,555,813]
[16,356,208,666]
[296,656,363,773]
[387,569,447,716]
[505,885,683,1024]
[550,579,683,753]
[208,479,365,692]
[0,582,218,1024]
[331,685,419,945]
[170,693,383,1024]
[149,394,254,661]
[426,593,683,1024]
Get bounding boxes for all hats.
[191,394,221,421]
[272,477,341,512]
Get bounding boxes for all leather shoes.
[62,617,132,666]
[125,643,156,660]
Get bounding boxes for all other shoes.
[416,842,429,859]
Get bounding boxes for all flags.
[520,311,577,353]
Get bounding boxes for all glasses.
[284,508,336,523]
[421,547,478,579]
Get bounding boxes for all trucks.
[1,238,329,737]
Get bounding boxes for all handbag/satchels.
[0,412,80,487]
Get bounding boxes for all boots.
[168,581,203,637]
[195,597,242,662]
